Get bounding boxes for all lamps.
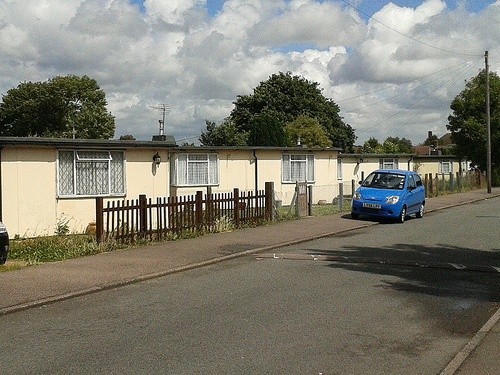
[154,152,161,168]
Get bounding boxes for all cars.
[0,221,9,266]
[351,169,427,223]
